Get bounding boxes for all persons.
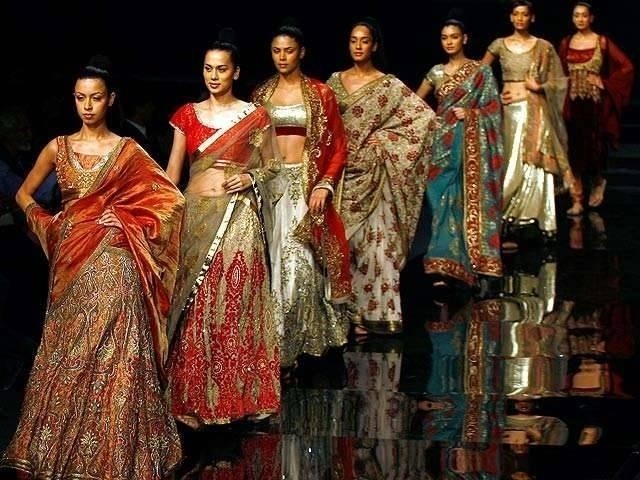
[0,65,184,480]
[324,19,436,334]
[163,41,288,432]
[416,18,506,301]
[556,0,635,216]
[479,0,578,240]
[249,24,352,370]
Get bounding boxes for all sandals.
[589,179,606,208]
